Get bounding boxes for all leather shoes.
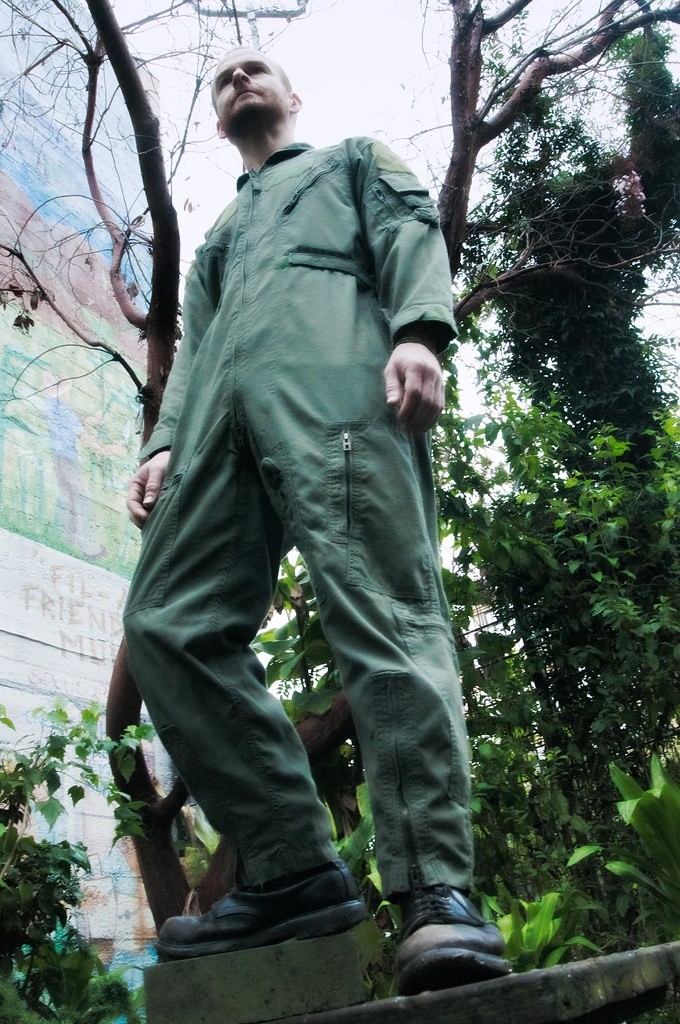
[395,884,512,993]
[153,856,368,956]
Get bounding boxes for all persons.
[122,48,514,997]
